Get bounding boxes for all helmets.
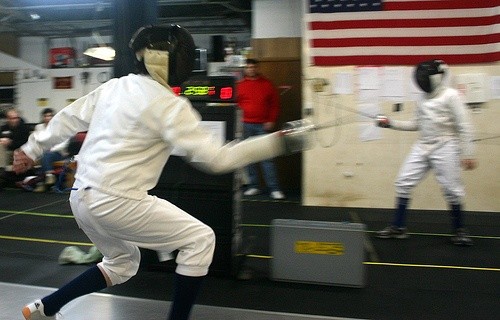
[415,59,448,94]
[130,23,196,89]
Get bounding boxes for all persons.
[0,108,28,189]
[29,108,70,193]
[10,23,289,320]
[234,58,285,202]
[375,56,477,248]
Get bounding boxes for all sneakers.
[15,173,56,192]
[21,299,57,320]
[271,191,285,199]
[376,225,411,239]
[243,188,262,195]
[451,229,474,246]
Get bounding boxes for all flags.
[303,1,500,68]
